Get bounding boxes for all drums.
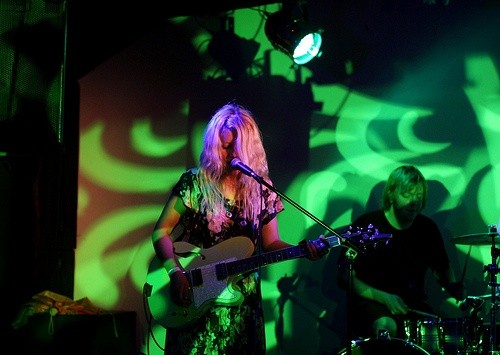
[404,317,485,355]
[337,327,430,355]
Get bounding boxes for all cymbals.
[450,226,500,245]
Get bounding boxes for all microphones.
[279,272,304,304]
[231,158,257,177]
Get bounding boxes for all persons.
[339,165,453,341]
[151,101,331,355]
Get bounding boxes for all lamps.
[264,10,326,66]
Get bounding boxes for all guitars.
[145,224,393,329]
